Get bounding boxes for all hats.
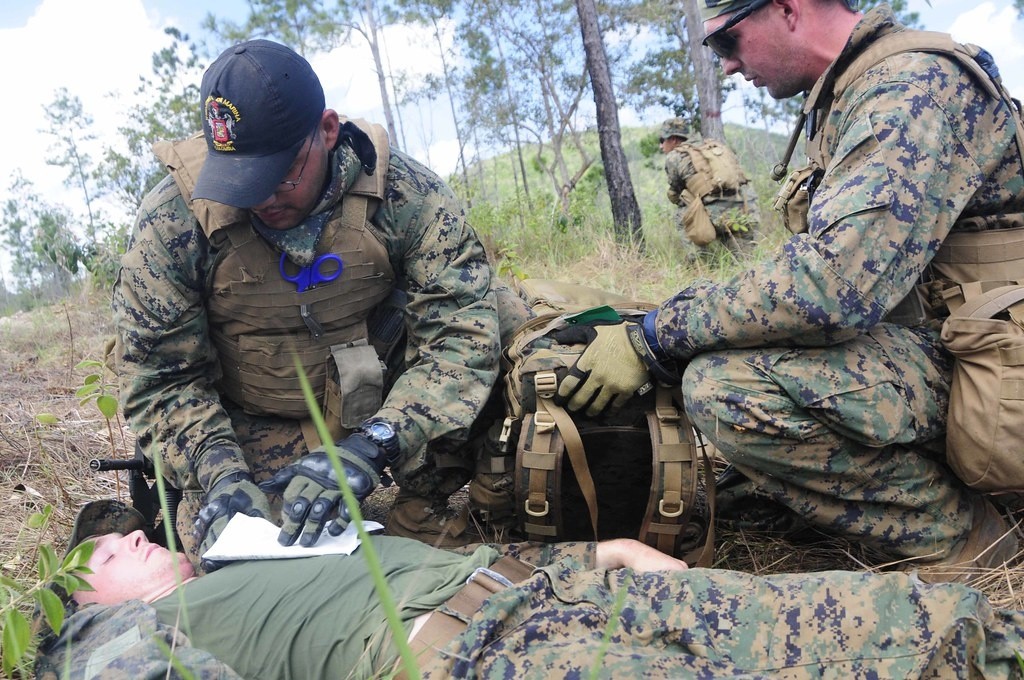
[697,0,759,23]
[661,119,690,139]
[33,500,146,634]
[189,38,324,208]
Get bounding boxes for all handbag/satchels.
[679,196,716,245]
[937,280,1024,490]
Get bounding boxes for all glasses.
[274,121,320,193]
[660,137,664,143]
[702,0,772,60]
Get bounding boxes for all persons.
[35,498,1024,680]
[552,0,1024,587]
[659,119,759,268]
[111,39,530,577]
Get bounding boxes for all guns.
[88,435,185,550]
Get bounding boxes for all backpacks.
[469,297,717,568]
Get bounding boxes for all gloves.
[258,431,394,547]
[551,317,682,419]
[191,472,271,573]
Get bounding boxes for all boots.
[385,486,510,549]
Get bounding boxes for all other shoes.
[897,495,1018,594]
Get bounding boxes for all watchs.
[354,422,400,466]
